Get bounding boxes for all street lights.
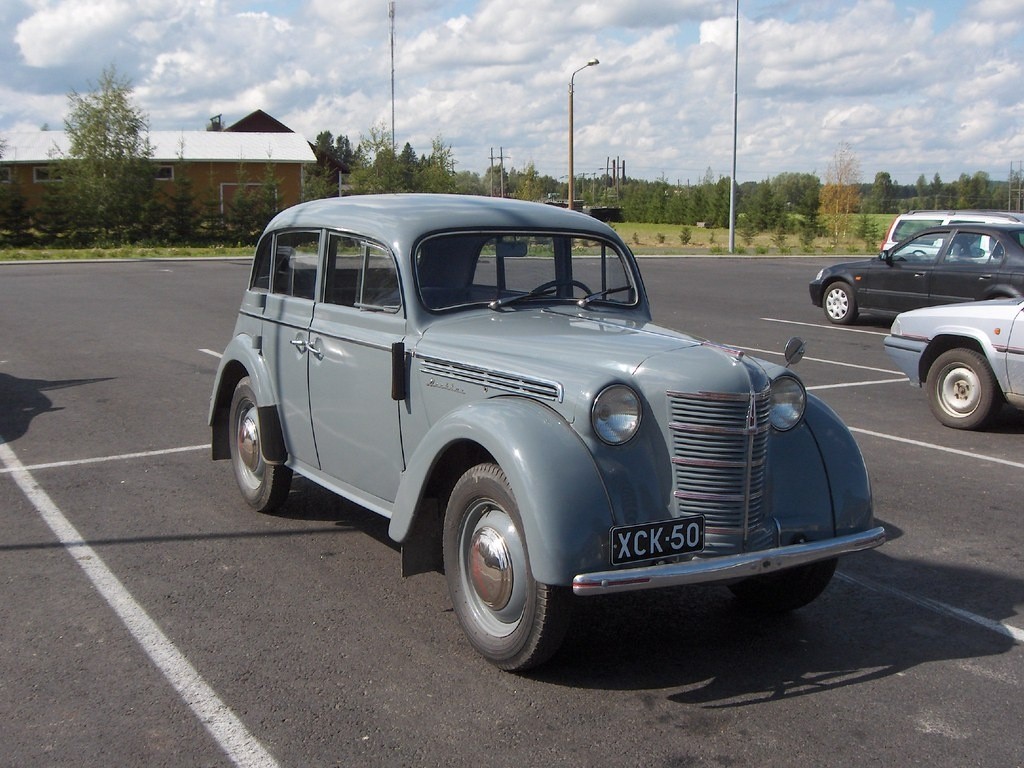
[566,55,600,208]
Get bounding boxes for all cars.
[809,223,1024,324]
[207,196,885,677]
[884,293,1024,430]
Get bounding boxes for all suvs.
[881,207,1024,255]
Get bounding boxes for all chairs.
[951,243,963,258]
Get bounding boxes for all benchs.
[326,286,447,309]
